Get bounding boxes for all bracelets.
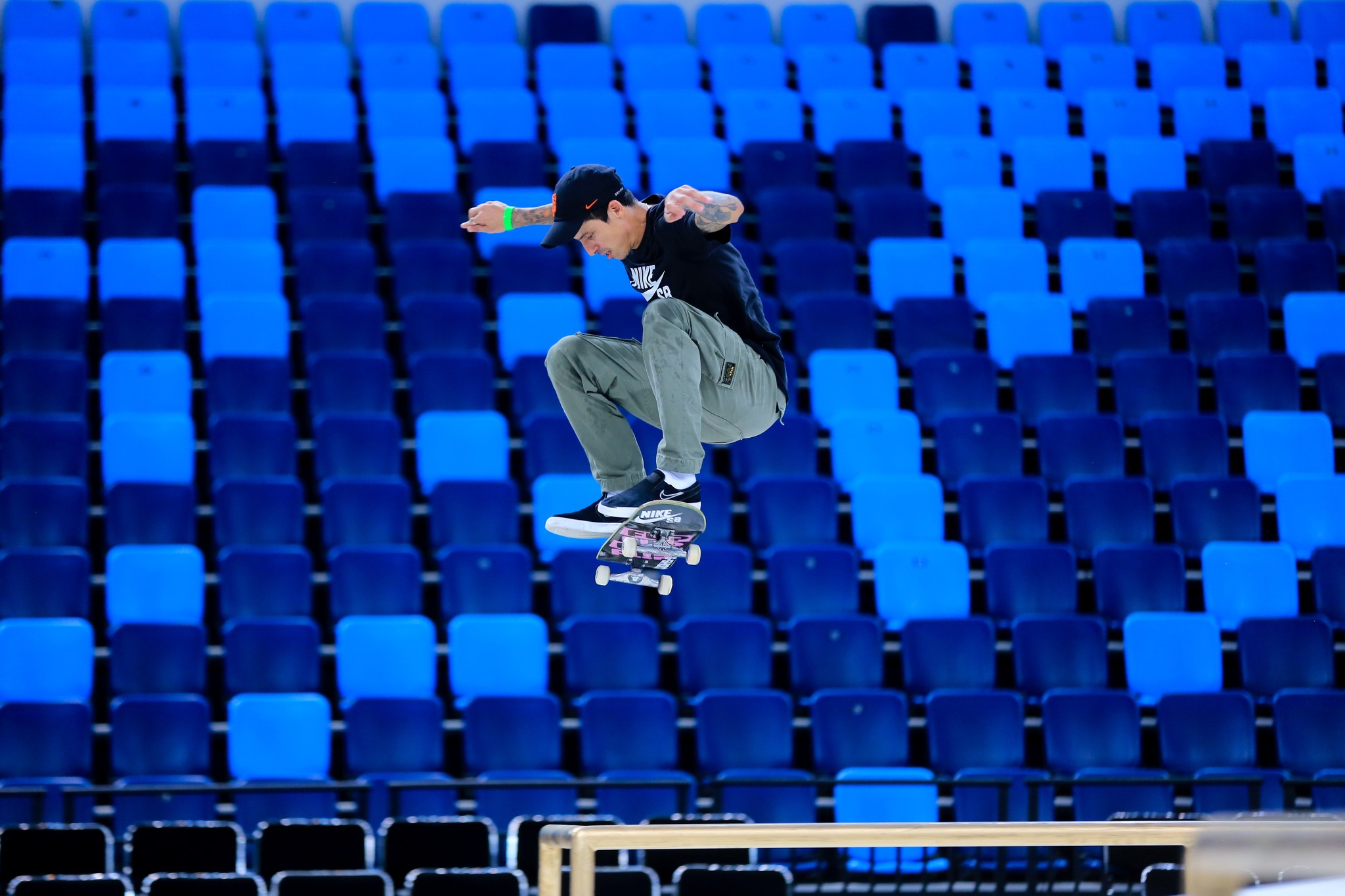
[503,206,516,231]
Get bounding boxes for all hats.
[540,165,625,249]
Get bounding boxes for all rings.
[470,217,477,225]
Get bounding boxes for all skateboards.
[595,500,707,594]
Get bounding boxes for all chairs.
[0,0,1345,896]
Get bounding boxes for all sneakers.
[597,470,702,518]
[545,492,628,539]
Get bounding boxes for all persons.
[460,166,788,539]
